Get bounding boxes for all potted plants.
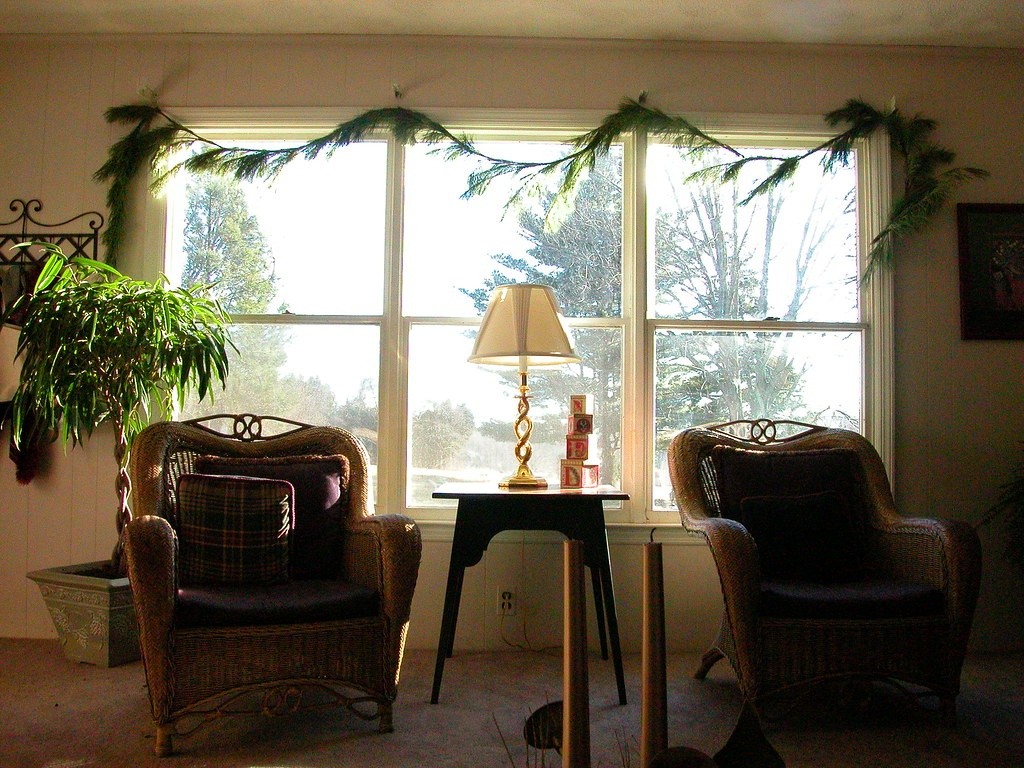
[1,241,242,667]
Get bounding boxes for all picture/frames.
[957,201,1024,341]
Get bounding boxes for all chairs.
[668,420,980,727]
[123,415,423,755]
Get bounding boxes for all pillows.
[711,442,878,558]
[177,473,296,588]
[741,490,876,585]
[194,452,350,584]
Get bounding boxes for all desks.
[429,483,631,707]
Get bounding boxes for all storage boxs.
[560,394,598,489]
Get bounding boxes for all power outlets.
[497,586,516,616]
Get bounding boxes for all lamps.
[467,284,580,490]
